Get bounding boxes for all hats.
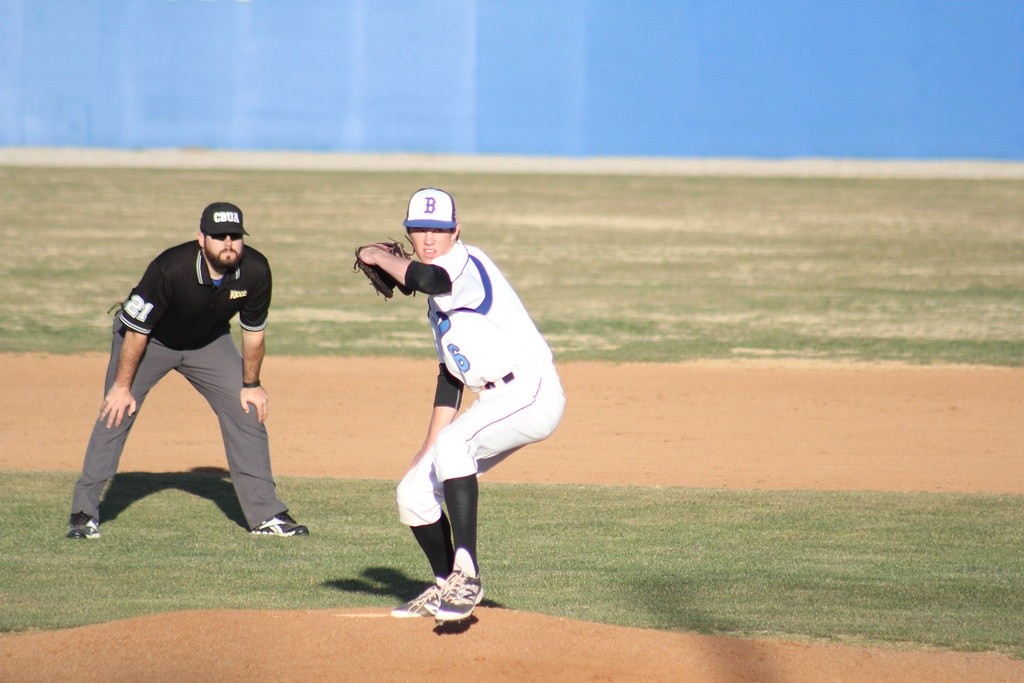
[403,188,457,229]
[200,202,249,236]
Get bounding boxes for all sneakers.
[434,570,484,623]
[250,513,308,536]
[66,511,101,538]
[391,576,447,618]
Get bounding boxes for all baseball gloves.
[350,233,415,302]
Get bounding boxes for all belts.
[484,372,514,389]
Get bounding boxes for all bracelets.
[243,380,260,388]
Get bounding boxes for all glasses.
[211,233,242,241]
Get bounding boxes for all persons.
[65,202,311,540]
[356,187,565,626]
